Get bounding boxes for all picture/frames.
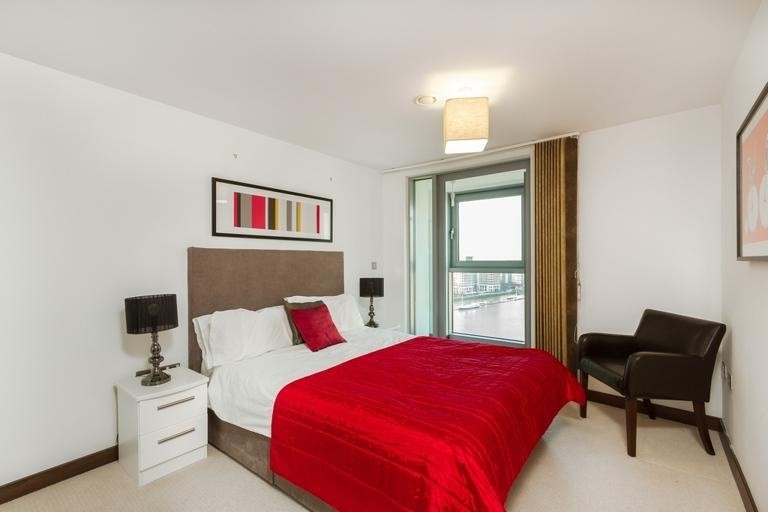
[736,81,768,266]
[211,176,333,242]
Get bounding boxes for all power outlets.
[721,360,733,390]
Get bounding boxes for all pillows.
[193,294,361,369]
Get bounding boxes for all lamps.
[124,293,179,385]
[360,277,384,328]
[442,96,489,155]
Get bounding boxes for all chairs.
[579,307,728,461]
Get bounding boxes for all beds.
[185,245,586,512]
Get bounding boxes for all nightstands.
[376,322,400,331]
[116,366,208,488]
